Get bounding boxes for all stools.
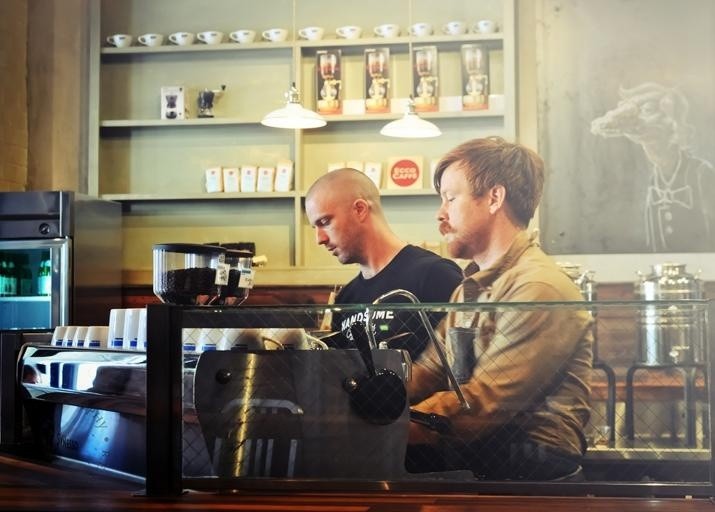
[537,461,586,483]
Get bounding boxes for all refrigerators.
[0,187,127,456]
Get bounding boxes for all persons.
[406,136,594,481]
[305,167,463,405]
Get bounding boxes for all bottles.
[0,250,51,296]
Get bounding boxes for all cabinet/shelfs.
[80,0,527,290]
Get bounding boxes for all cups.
[407,22,434,36]
[228,30,257,43]
[334,25,362,39]
[298,26,325,39]
[439,21,469,35]
[196,31,225,43]
[264,28,289,42]
[50,325,108,348]
[168,31,195,44]
[471,19,499,35]
[203,160,294,193]
[107,29,135,47]
[134,32,165,45]
[107,304,330,369]
[371,23,401,38]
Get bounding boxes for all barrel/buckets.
[558,262,598,362]
[633,264,709,368]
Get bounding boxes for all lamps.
[376,0,445,140]
[258,0,329,132]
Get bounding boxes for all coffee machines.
[223,249,253,309]
[14,288,471,490]
[153,241,224,306]
